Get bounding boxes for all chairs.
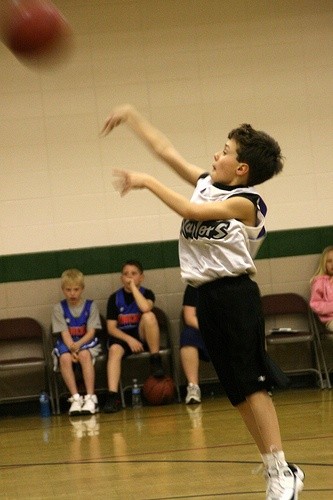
[48,315,121,414]
[259,293,327,391]
[310,303,333,390]
[106,306,182,409]
[0,318,56,415]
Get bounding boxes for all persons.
[99,104,304,500]
[105,259,162,413]
[309,246,333,333]
[178,284,211,403]
[52,268,102,415]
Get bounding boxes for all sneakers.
[81,394,100,415]
[264,464,305,500]
[67,393,84,416]
[150,354,165,378]
[103,392,121,413]
[185,384,201,405]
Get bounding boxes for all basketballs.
[142,374,176,405]
[0,0,77,72]
